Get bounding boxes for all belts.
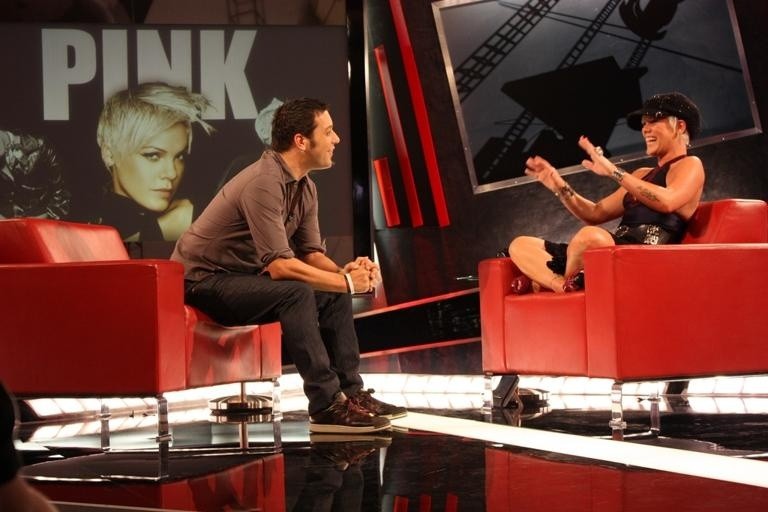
[614,223,670,245]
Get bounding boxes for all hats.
[626,93,700,139]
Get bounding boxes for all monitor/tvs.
[430,0,763,197]
[0,1,375,297]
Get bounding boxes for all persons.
[508,92,706,293]
[171,97,408,435]
[293,431,394,512]
[0,381,59,511]
[68,80,220,244]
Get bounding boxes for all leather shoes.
[563,270,584,293]
[510,276,547,294]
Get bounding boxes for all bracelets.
[553,183,573,199]
[345,272,356,295]
[611,167,625,182]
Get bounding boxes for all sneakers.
[349,388,406,419]
[309,434,393,471]
[310,393,391,433]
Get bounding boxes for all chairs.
[477,196,768,442]
[1,216,284,442]
